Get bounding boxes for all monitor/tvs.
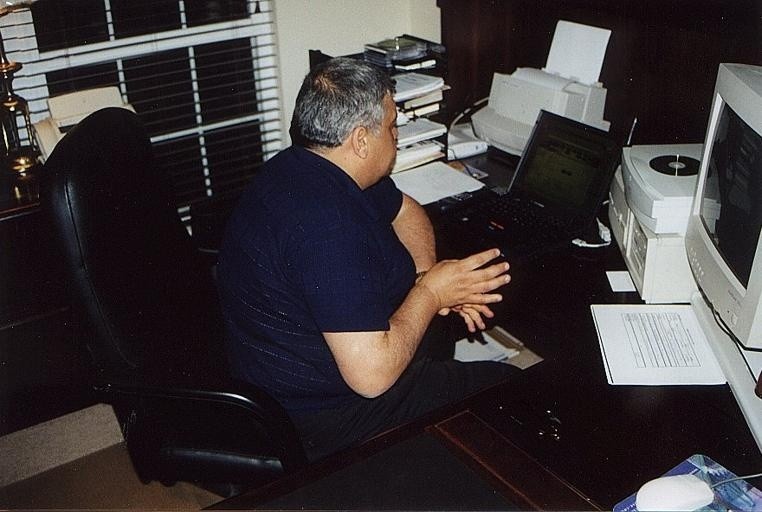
[439,109,624,263]
[682,60,761,452]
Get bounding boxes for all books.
[396,119,447,148]
[403,103,440,121]
[395,89,442,109]
[392,141,441,169]
[390,73,444,102]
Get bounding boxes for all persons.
[218,59,522,463]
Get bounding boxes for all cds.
[650,154,701,177]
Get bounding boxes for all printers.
[471,67,611,169]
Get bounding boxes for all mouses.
[635,474,714,512]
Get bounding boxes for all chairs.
[45,106,304,500]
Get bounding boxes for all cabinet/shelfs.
[1,201,104,437]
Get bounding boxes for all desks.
[198,186,762,512]
